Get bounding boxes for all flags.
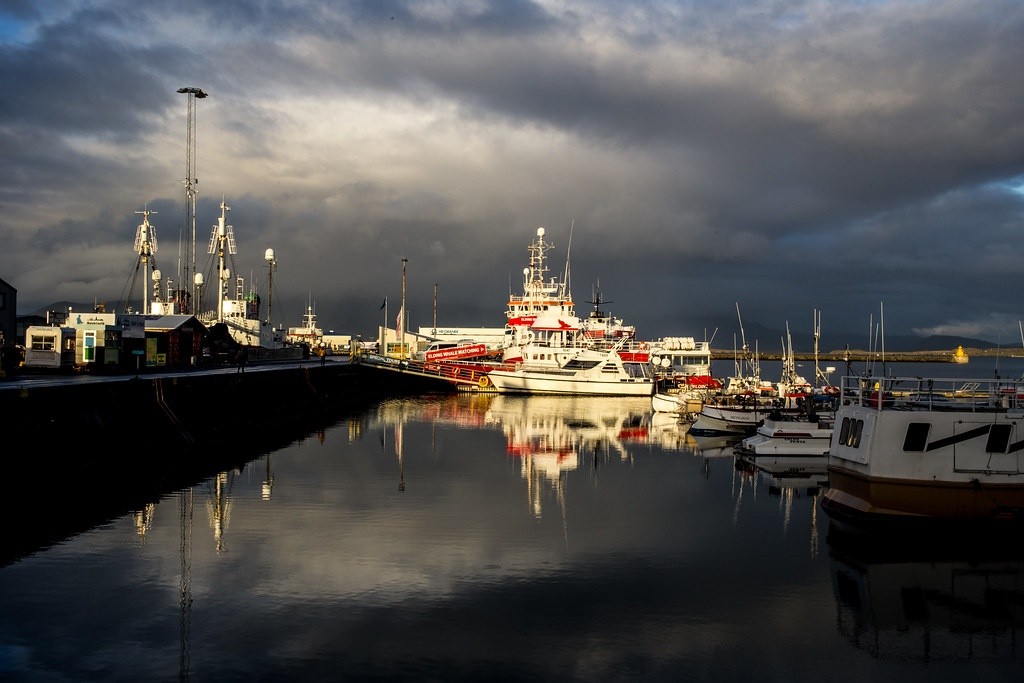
[379,296,389,311]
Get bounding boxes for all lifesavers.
[478,376,489,388]
[638,342,647,350]
[452,366,460,375]
[424,363,430,370]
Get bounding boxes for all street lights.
[176,86,209,315]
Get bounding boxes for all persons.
[235,347,247,374]
[318,345,326,368]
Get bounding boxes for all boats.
[735,449,830,490]
[286,306,323,355]
[686,429,749,458]
[819,488,1023,561]
[651,411,699,427]
[485,216,656,395]
[644,300,1023,520]
[195,192,276,349]
[489,393,654,547]
